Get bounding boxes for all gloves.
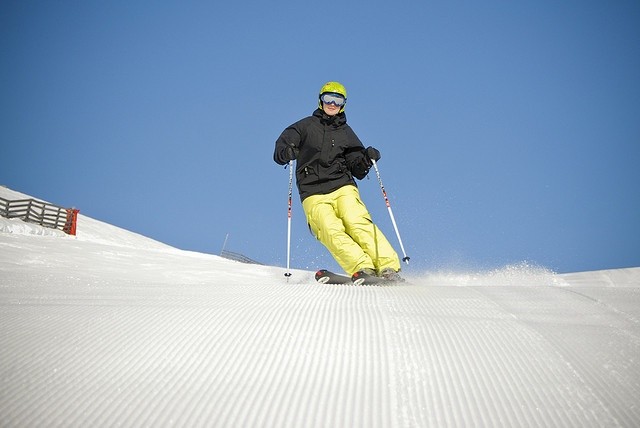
[284,146,300,160]
[367,147,382,166]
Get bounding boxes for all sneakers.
[352,268,377,281]
[379,267,405,280]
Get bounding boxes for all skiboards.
[315,269,413,286]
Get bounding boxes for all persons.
[273,81,402,280]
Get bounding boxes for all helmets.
[317,81,348,114]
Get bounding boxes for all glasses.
[321,93,346,107]
